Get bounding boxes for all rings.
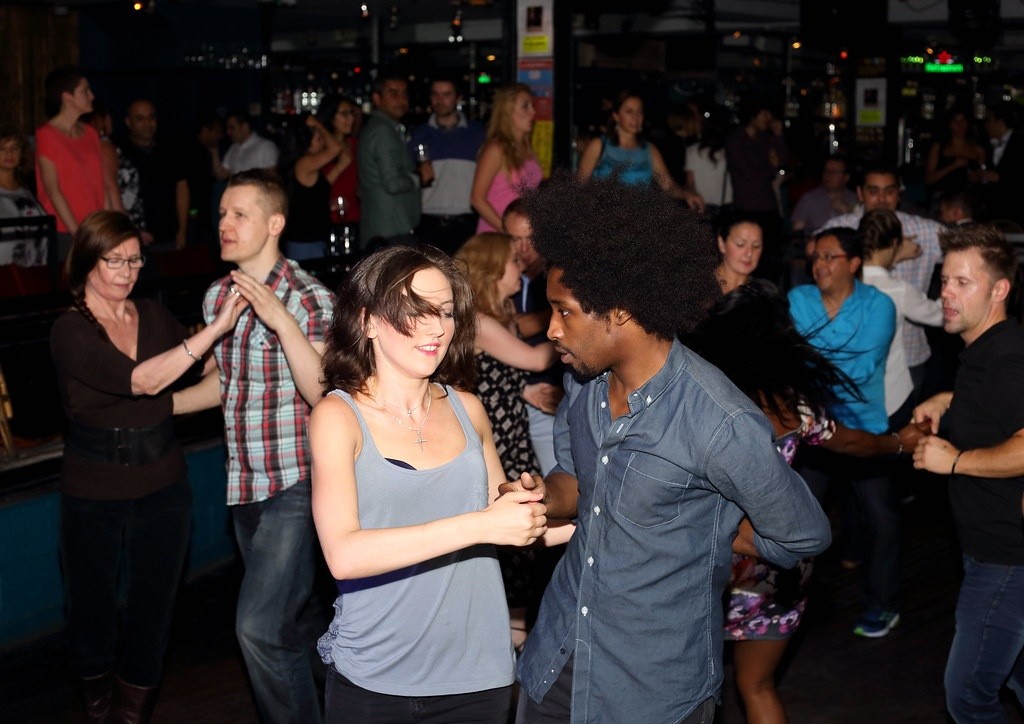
[230,288,237,294]
[236,292,241,296]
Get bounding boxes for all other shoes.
[852,601,901,637]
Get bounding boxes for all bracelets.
[951,450,962,474]
[183,339,202,361]
[892,433,904,454]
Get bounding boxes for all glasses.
[101,258,147,270]
[814,253,850,263]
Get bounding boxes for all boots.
[81,671,112,724]
[112,675,159,724]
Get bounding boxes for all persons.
[171,167,332,724]
[688,283,932,723]
[908,227,1024,724]
[0,65,1024,652]
[49,208,249,724]
[494,177,832,723]
[306,240,547,723]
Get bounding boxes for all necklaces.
[388,402,425,415]
[367,377,432,451]
